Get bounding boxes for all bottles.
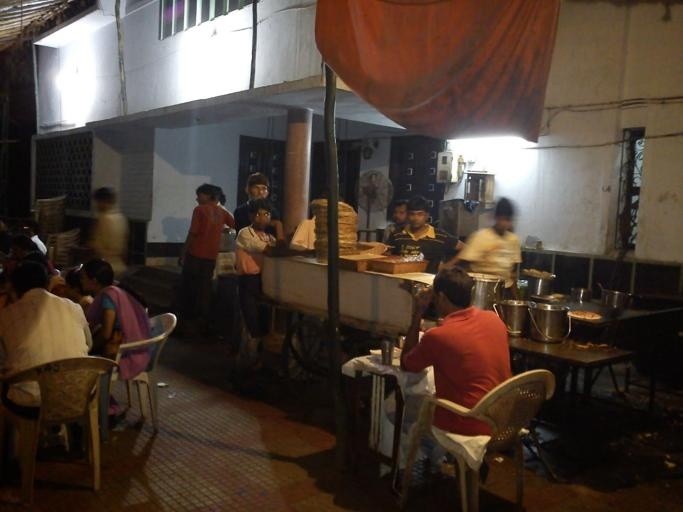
[380,339,392,365]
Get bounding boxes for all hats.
[247,173,270,186]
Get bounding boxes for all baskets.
[312,198,359,263]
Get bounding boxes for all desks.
[556,298,682,398]
[497,320,657,460]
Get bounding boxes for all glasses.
[253,211,271,217]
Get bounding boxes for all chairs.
[99,313,178,442]
[394,369,556,512]
[28,193,84,281]
[0,356,120,509]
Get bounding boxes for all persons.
[390,268,512,499]
[179,172,523,341]
[0,260,96,463]
[0,186,157,429]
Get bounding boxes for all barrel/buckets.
[527,303,572,344]
[492,300,531,339]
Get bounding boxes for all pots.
[464,271,571,344]
[599,290,642,313]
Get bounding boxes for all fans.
[354,170,394,243]
